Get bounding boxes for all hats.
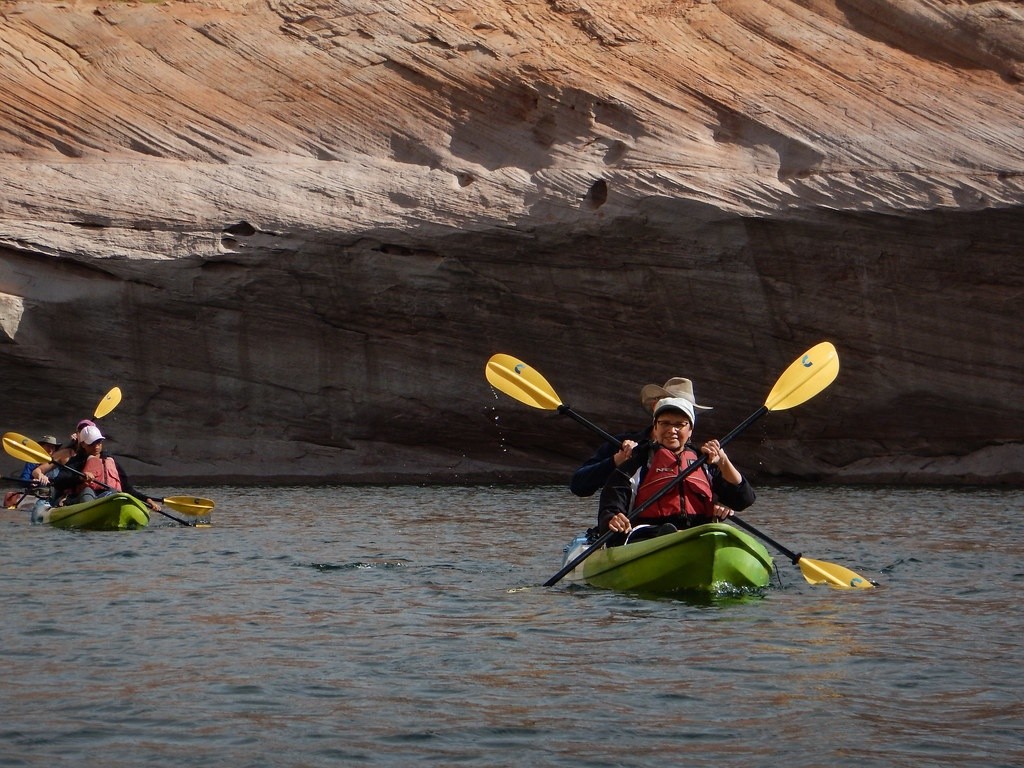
[37,435,62,451]
[640,376,714,415]
[75,419,97,432]
[64,443,78,452]
[653,397,695,430]
[77,426,106,445]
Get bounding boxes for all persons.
[570,377,756,548]
[20,419,162,513]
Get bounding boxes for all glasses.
[95,440,103,445]
[657,420,689,431]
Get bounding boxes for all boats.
[565,521,774,599]
[32,491,151,529]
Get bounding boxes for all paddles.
[8,386,122,510]
[0,430,195,528]
[1,475,216,517]
[484,351,875,589]
[539,339,841,588]
